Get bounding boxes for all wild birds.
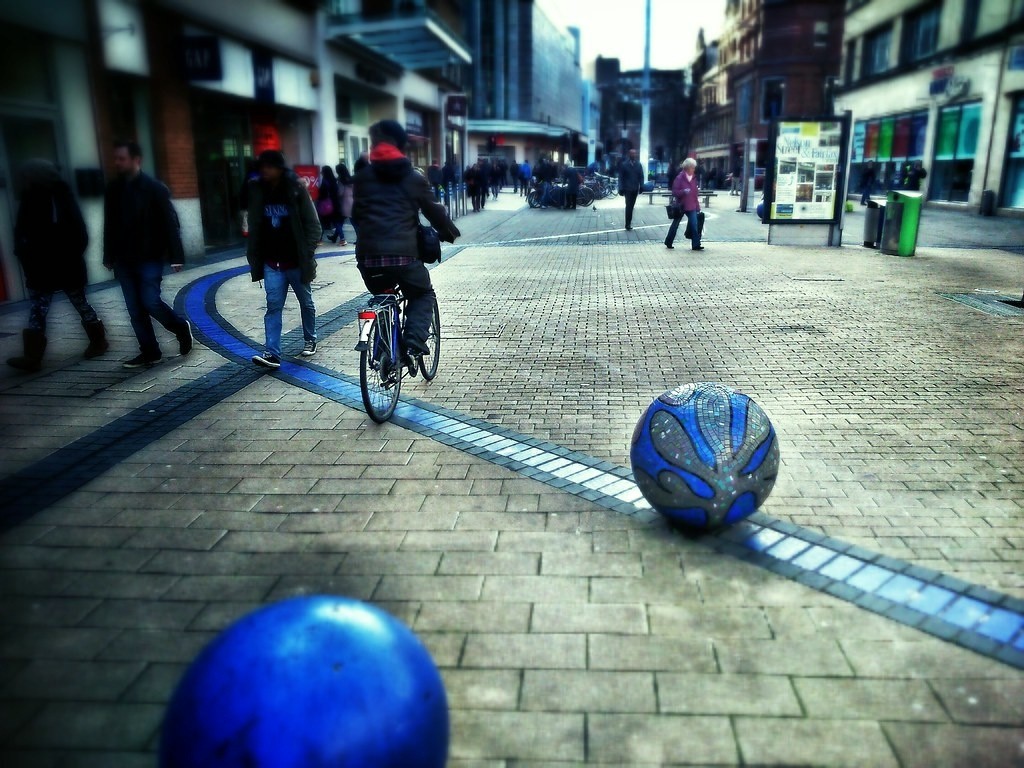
[593,205,598,212]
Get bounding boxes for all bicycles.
[527,172,618,207]
[345,231,441,423]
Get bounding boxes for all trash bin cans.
[980,190,993,216]
[880,189,923,257]
[864,200,887,247]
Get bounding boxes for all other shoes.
[693,246,704,250]
[667,245,674,249]
[625,225,633,230]
[473,208,480,212]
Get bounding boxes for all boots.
[6,328,47,371]
[81,319,109,359]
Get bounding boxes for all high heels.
[327,234,337,243]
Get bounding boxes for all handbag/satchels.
[667,194,683,219]
[316,198,333,215]
[575,172,583,185]
[416,224,441,264]
[685,211,705,240]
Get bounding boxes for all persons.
[729,160,742,196]
[103,139,193,369]
[5,160,109,369]
[315,164,353,246]
[246,149,323,368]
[462,158,508,212]
[428,159,456,206]
[351,120,461,367]
[617,149,644,229]
[509,159,584,209]
[667,164,716,190]
[664,158,704,250]
[904,161,927,190]
[859,160,876,206]
[354,152,369,174]
[214,158,238,220]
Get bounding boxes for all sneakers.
[177,320,194,356]
[252,355,280,368]
[122,353,162,368]
[302,342,317,356]
[337,239,348,246]
[406,337,430,355]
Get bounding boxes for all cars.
[740,168,766,188]
